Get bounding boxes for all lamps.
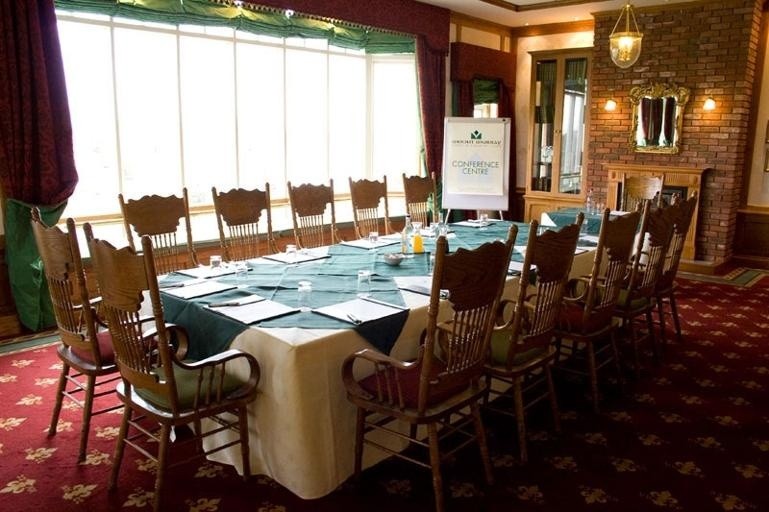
[608,0,645,70]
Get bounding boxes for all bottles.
[584,189,606,217]
[400,213,425,254]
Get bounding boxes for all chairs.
[539,170,699,369]
[211,184,282,263]
[608,188,700,363]
[119,188,202,276]
[288,180,342,250]
[349,175,396,240]
[29,205,161,465]
[479,212,584,467]
[552,199,644,418]
[341,224,518,510]
[402,171,438,230]
[83,222,260,510]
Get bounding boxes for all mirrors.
[628,81,692,154]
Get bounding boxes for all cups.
[203,245,436,312]
[415,213,595,242]
[367,230,379,245]
